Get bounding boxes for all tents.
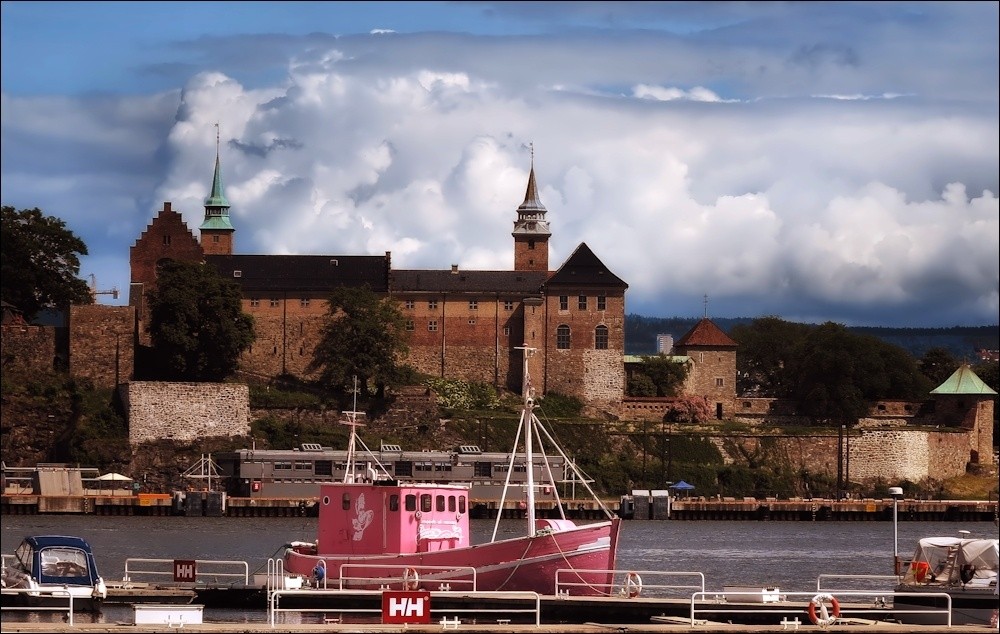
[668,480,695,501]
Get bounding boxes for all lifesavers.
[402,568,418,589]
[622,573,642,597]
[810,593,839,626]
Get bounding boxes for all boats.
[279,342,626,596]
[893,535,999,625]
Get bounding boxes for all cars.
[10,534,108,599]
